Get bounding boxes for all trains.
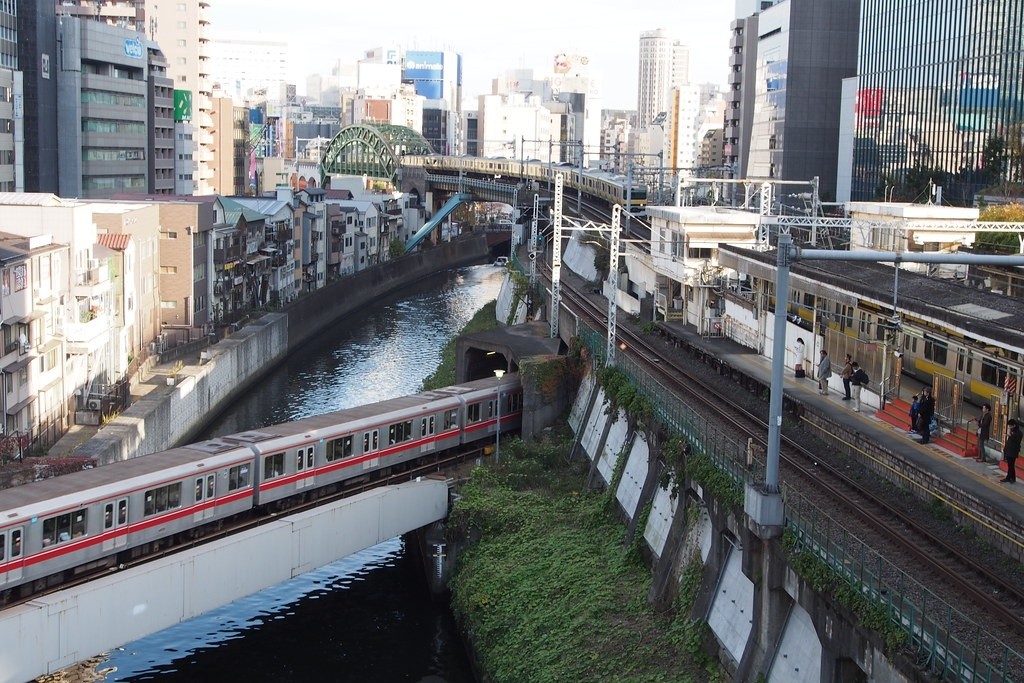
[348,152,649,218]
[751,278,1024,425]
[0,371,522,610]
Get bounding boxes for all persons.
[1000,419,1023,483]
[816,350,832,395]
[849,362,862,412]
[917,387,935,444]
[42,524,83,548]
[269,463,280,478]
[794,338,805,375]
[973,403,992,462]
[909,396,920,433]
[839,354,853,400]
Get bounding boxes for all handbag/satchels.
[915,417,925,430]
[796,369,805,378]
[929,417,937,431]
[819,380,828,389]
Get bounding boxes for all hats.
[912,395,918,400]
[851,362,858,367]
[923,387,933,393]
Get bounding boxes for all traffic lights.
[537,236,544,250]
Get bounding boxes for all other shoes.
[819,390,829,396]
[842,397,851,401]
[853,408,860,413]
[920,440,927,444]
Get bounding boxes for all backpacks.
[860,373,869,386]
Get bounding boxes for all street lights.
[494,369,505,463]
